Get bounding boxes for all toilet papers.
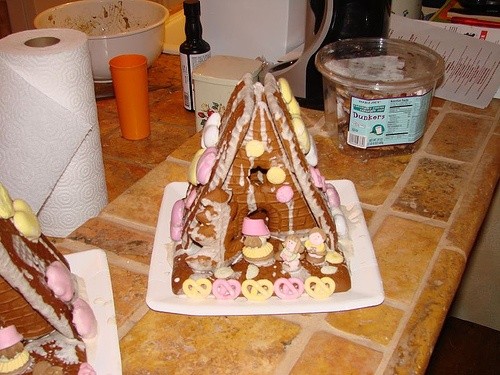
[1,26,108,237]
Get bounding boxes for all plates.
[64,249,121,375]
[146,180,384,315]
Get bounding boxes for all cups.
[109,54,150,141]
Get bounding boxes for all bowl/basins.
[34,0,169,83]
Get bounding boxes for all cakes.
[170,73,352,300]
[0,183,98,375]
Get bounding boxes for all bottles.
[179,0,212,111]
